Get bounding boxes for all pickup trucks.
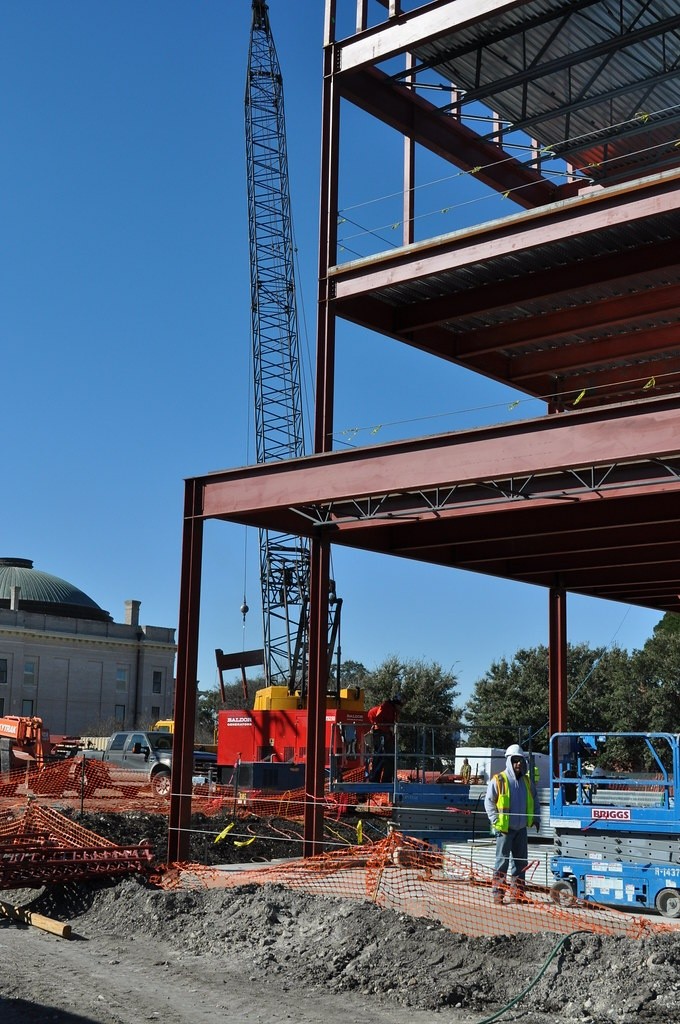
[76,729,218,803]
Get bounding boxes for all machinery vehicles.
[0,714,85,783]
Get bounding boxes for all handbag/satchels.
[364,724,395,752]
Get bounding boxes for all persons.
[368,695,402,783]
[484,744,541,904]
[461,759,471,784]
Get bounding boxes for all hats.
[505,744,530,760]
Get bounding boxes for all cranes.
[244,0,364,712]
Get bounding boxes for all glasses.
[511,759,525,763]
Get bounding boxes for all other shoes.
[510,897,534,904]
[494,898,503,904]
